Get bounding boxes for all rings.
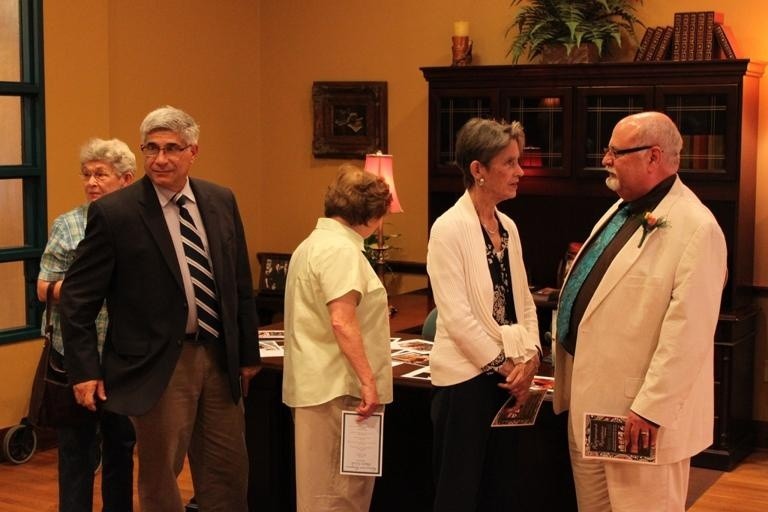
[642,431,649,435]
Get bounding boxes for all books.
[633,11,736,61]
[678,134,724,171]
[530,291,549,300]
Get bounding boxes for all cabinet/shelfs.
[533,304,762,471]
[419,58,768,307]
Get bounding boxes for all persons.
[552,111,727,511]
[263,259,287,290]
[539,228,589,294]
[36,138,136,512]
[59,105,260,512]
[282,162,394,512]
[424,117,543,512]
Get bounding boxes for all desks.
[243,293,564,406]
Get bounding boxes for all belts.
[173,328,222,345]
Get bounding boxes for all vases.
[501,1,645,64]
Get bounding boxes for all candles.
[452,17,470,36]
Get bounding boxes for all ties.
[171,193,221,349]
[555,202,633,348]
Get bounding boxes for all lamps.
[360,151,402,286]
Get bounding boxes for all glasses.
[139,143,193,154]
[605,145,664,156]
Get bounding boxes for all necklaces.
[483,217,498,233]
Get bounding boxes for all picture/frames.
[310,79,389,160]
[255,252,292,294]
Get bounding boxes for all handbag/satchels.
[27,280,79,434]
